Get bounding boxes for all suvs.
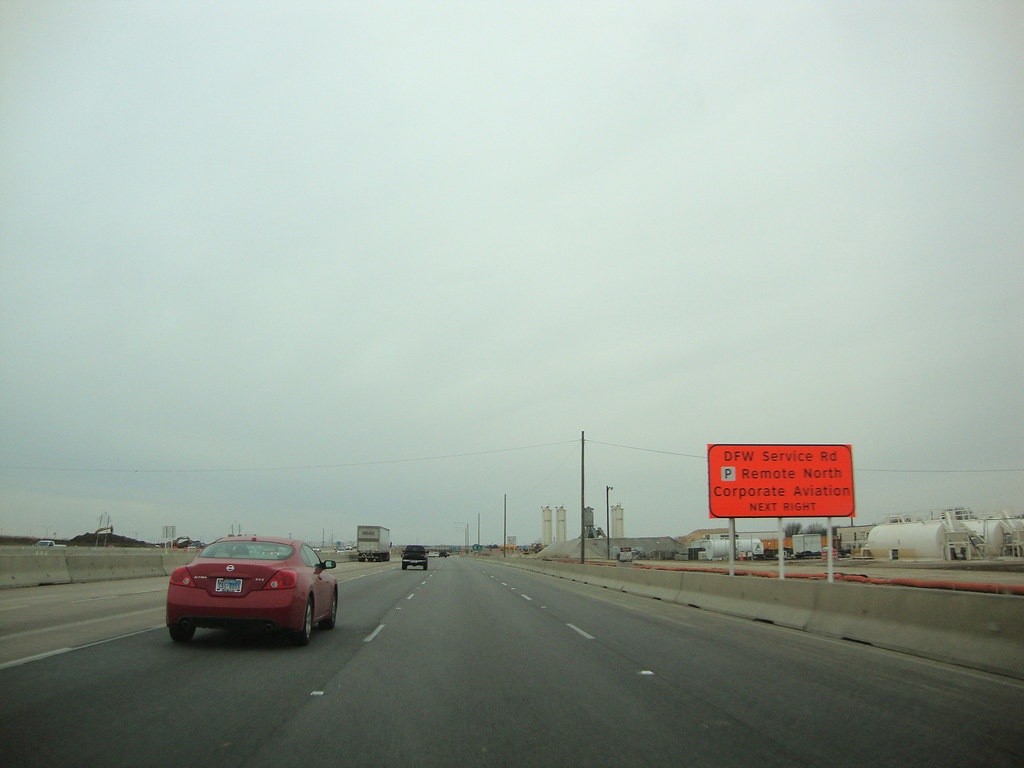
[401,544,429,570]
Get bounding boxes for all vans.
[438,549,447,558]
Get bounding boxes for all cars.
[345,544,353,550]
[165,536,339,645]
[30,541,55,547]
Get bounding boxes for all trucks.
[357,525,392,561]
